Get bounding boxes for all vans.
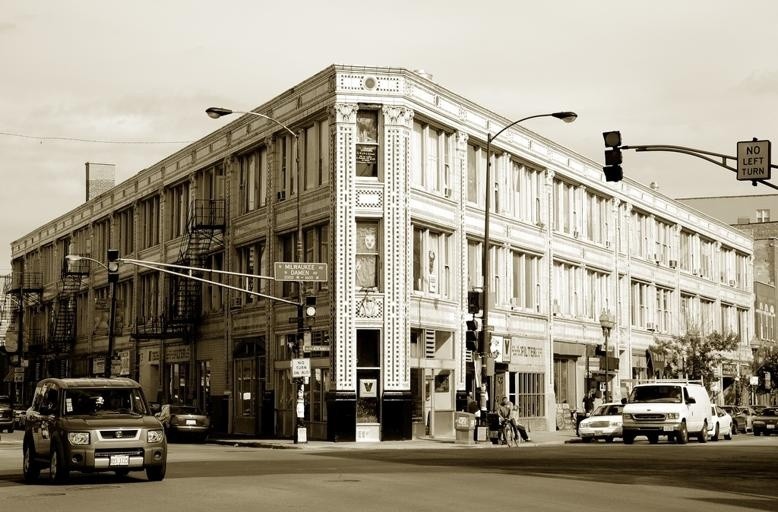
[619,382,712,442]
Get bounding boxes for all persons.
[498,397,532,444]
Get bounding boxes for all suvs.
[0,394,14,433]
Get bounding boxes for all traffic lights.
[305,295,317,329]
[107,249,119,283]
[465,319,478,352]
[601,131,625,182]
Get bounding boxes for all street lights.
[66,255,119,378]
[598,309,616,403]
[749,332,762,406]
[479,110,578,409]
[205,105,309,444]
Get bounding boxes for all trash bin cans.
[576,412,589,437]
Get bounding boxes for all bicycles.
[500,418,522,448]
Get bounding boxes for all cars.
[147,401,210,444]
[21,376,168,483]
[10,404,27,431]
[711,405,778,440]
[577,402,625,443]
